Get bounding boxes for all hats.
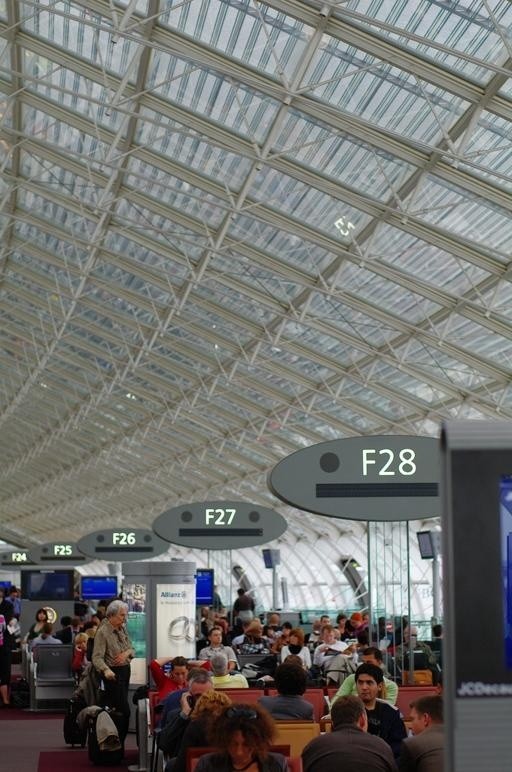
[351,613,362,621]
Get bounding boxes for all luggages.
[88,707,125,766]
[63,697,87,748]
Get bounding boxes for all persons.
[92,597,135,750]
[0,579,125,702]
[146,605,447,771]
[232,587,258,624]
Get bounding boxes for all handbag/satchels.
[9,677,30,708]
[86,637,94,661]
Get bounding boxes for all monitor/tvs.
[80,575,118,598]
[20,569,74,600]
[415,530,435,559]
[262,548,273,569]
[196,568,214,605]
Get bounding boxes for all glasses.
[224,706,257,720]
